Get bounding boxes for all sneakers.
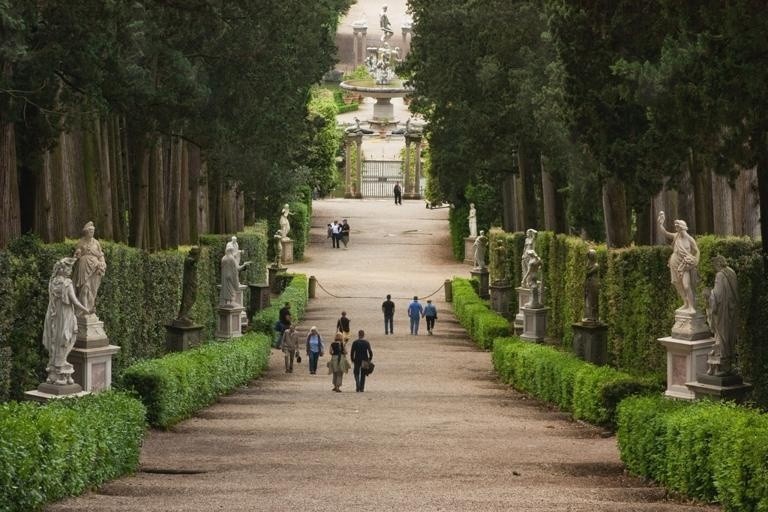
[385,330,434,336]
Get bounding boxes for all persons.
[218,247,239,306]
[377,4,394,42]
[41,256,91,383]
[466,202,479,239]
[177,245,202,322]
[304,326,325,374]
[655,210,701,315]
[335,311,350,347]
[520,228,537,288]
[492,239,507,280]
[364,44,403,84]
[349,330,374,392]
[392,180,402,205]
[274,202,296,237]
[582,249,601,321]
[273,301,293,351]
[406,296,422,335]
[224,234,245,264]
[71,220,107,314]
[341,219,350,248]
[328,220,342,248]
[702,254,740,360]
[279,323,301,374]
[422,299,438,335]
[520,248,542,287]
[380,294,396,336]
[469,229,488,271]
[325,333,351,392]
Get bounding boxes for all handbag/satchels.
[275,320,289,333]
[295,352,301,363]
[366,361,375,376]
[342,329,352,339]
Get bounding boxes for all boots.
[285,368,365,392]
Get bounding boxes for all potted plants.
[343,90,352,105]
[377,116,390,138]
